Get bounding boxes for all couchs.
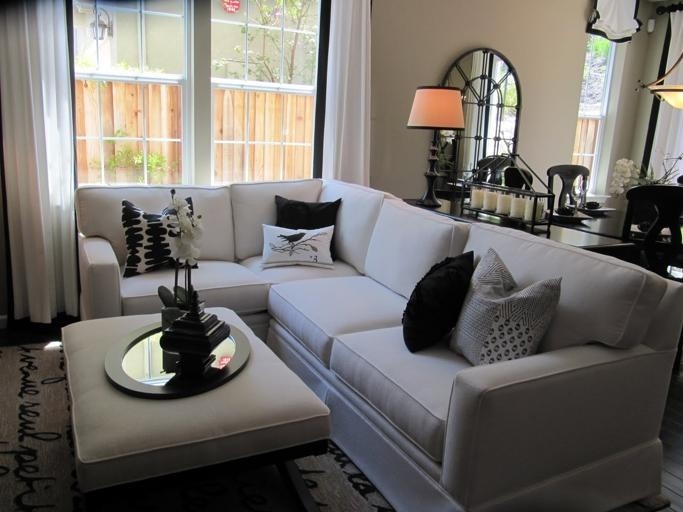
[75,175,678,510]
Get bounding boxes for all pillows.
[447,246,562,368]
[261,224,335,270]
[117,196,201,278]
[273,194,342,260]
[402,250,476,354]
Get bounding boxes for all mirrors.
[104,315,252,401]
[428,47,521,197]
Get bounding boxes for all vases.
[159,303,204,335]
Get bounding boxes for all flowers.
[608,152,683,196]
[157,185,204,310]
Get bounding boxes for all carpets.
[0,341,395,511]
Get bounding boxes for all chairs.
[621,185,683,283]
[546,165,590,210]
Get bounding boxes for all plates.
[545,209,592,220]
[571,203,617,215]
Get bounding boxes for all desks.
[456,212,637,261]
[553,214,683,249]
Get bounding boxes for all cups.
[468,189,546,220]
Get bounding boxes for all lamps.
[405,86,466,206]
[90,8,114,44]
[631,0,683,109]
